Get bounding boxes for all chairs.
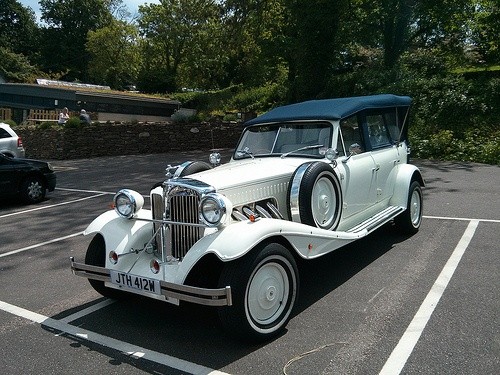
[318,128,330,148]
[277,127,296,152]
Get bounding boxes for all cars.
[0,152,57,208]
[69,94,427,337]
[0,121,25,159]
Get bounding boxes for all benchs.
[337,125,380,155]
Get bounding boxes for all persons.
[58,107,70,126]
[79,109,91,124]
